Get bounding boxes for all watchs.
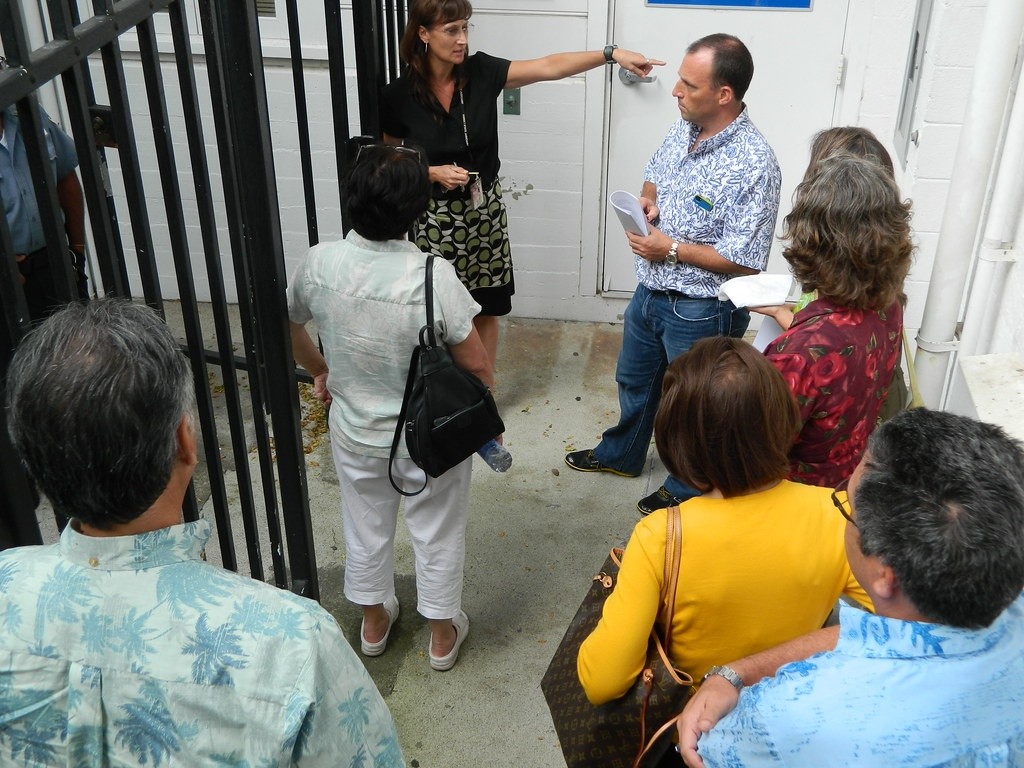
[666,240,680,265]
[700,664,744,690]
[604,44,618,64]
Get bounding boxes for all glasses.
[831,479,858,528]
[434,23,475,36]
[355,143,421,164]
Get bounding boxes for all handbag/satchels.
[541,505,697,768]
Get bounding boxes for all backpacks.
[388,254,505,496]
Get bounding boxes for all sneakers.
[565,449,641,478]
[636,486,684,516]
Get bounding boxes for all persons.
[676,404,1024,768]
[0,297,406,768]
[384,0,667,389]
[288,138,494,671]
[564,35,910,704]
[0,58,89,547]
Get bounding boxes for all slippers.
[360,595,400,656]
[428,610,469,672]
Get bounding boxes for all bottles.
[476,441,512,474]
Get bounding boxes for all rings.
[646,57,650,65]
